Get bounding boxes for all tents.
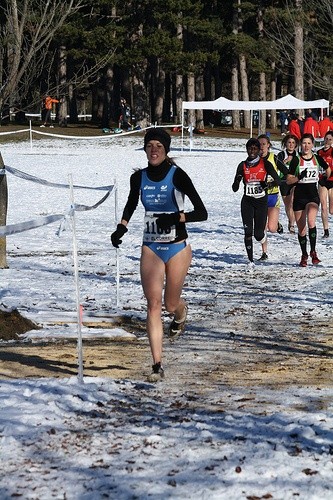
[181,94,329,151]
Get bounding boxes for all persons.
[286,134,331,267]
[277,109,333,238]
[40,96,61,128]
[232,138,280,266]
[257,135,288,260]
[111,128,208,383]
[118,98,133,130]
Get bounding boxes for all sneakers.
[168,301,188,338]
[148,361,164,381]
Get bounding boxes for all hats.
[144,128,172,154]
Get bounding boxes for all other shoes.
[310,251,321,264]
[50,126,54,128]
[277,222,283,235]
[288,221,295,233]
[321,235,329,239]
[257,253,268,261]
[39,125,46,128]
[300,255,308,267]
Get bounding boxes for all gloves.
[259,180,269,190]
[232,182,239,191]
[111,224,128,248]
[323,173,327,181]
[156,212,180,229]
[298,168,307,180]
